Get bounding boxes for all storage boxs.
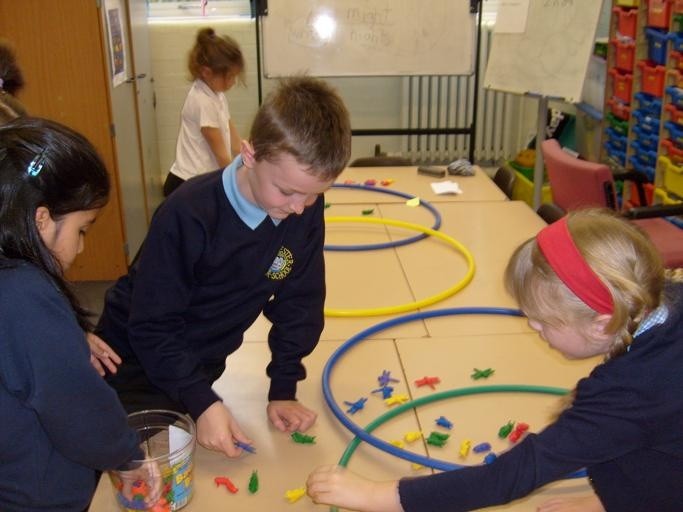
[637,59,666,97]
[662,138,683,166]
[664,103,683,127]
[663,120,683,150]
[664,86,683,110]
[654,187,683,206]
[631,109,660,134]
[672,14,683,34]
[643,26,669,65]
[670,50,683,73]
[628,155,655,182]
[629,139,657,167]
[631,125,659,151]
[633,91,662,116]
[573,102,604,163]
[607,113,629,136]
[612,6,638,40]
[582,53,607,113]
[611,38,635,71]
[602,140,627,166]
[646,0,671,29]
[667,68,683,88]
[604,126,627,152]
[610,68,633,103]
[606,99,630,121]
[668,32,683,52]
[657,155,683,200]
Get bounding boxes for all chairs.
[536,202,567,225]
[87,339,433,512]
[493,165,517,198]
[539,137,683,270]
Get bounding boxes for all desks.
[378,199,550,338]
[243,204,428,341]
[394,332,611,512]
[323,164,510,205]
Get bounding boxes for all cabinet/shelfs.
[600,0,683,229]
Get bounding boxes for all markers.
[525,92,543,98]
[546,96,564,101]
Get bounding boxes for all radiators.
[399,24,513,164]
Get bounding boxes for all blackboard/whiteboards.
[481,0,604,105]
[257,0,477,80]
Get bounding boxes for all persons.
[162,26,245,197]
[0,117,162,512]
[92,78,352,458]
[306,207,683,512]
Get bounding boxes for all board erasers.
[417,166,447,179]
